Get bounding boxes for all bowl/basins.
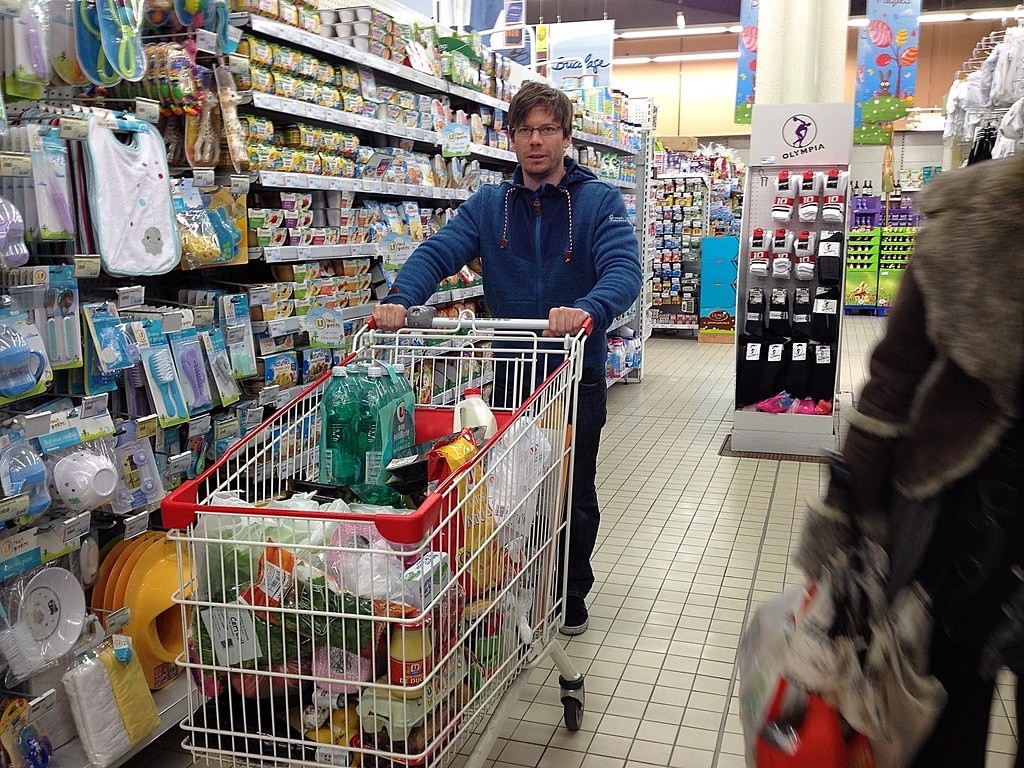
[54,449,119,512]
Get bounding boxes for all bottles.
[260,688,345,714]
[854,181,859,196]
[896,180,902,198]
[319,363,415,491]
[868,180,873,196]
[891,180,896,198]
[605,326,642,378]
[0,325,46,397]
[862,180,868,196]
[189,700,353,768]
[9,448,52,515]
[850,181,853,192]
[453,388,498,476]
[0,197,29,268]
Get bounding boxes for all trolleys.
[159,306,591,768]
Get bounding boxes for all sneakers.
[557,597,589,634]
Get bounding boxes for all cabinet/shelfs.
[230,12,640,389]
[651,175,712,337]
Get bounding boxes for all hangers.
[954,18,1024,128]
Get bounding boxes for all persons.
[373,82,642,635]
[794,154,1024,768]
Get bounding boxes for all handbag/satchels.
[738,582,876,768]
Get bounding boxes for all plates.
[17,568,87,661]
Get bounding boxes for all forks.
[43,288,57,361]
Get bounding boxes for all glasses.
[512,124,563,138]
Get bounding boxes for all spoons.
[57,289,76,358]
